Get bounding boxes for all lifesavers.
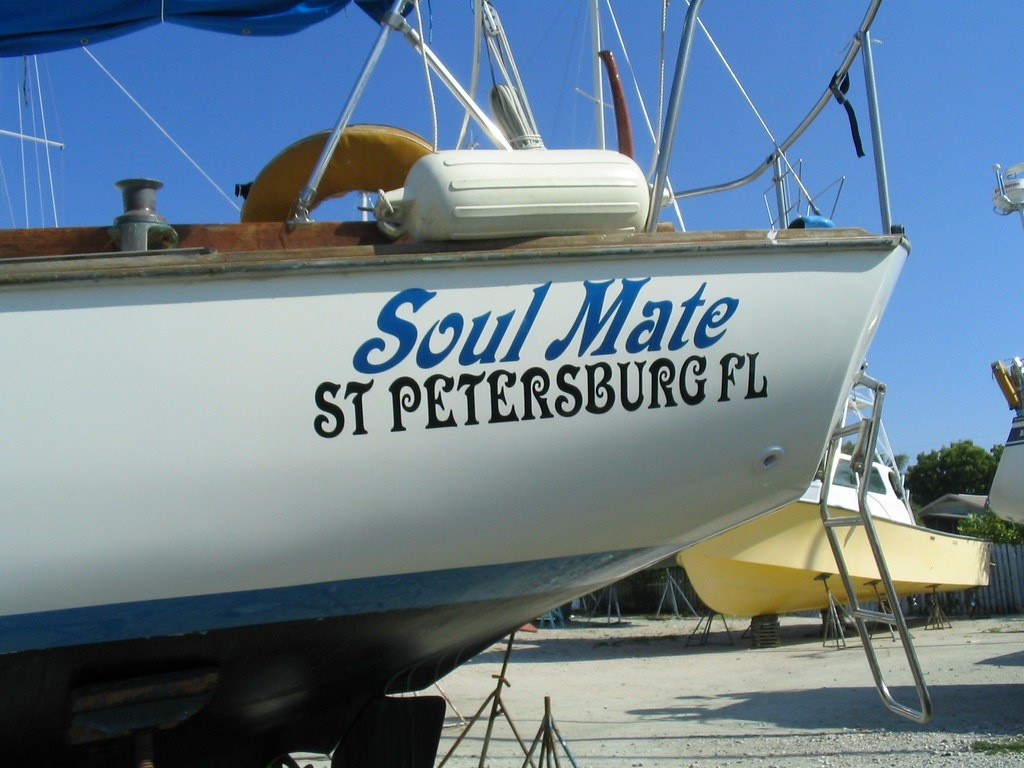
[989,360,1022,409]
[237,120,440,227]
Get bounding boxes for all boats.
[988,162,1024,526]
[0,1,932,767]
[676,386,994,616]
